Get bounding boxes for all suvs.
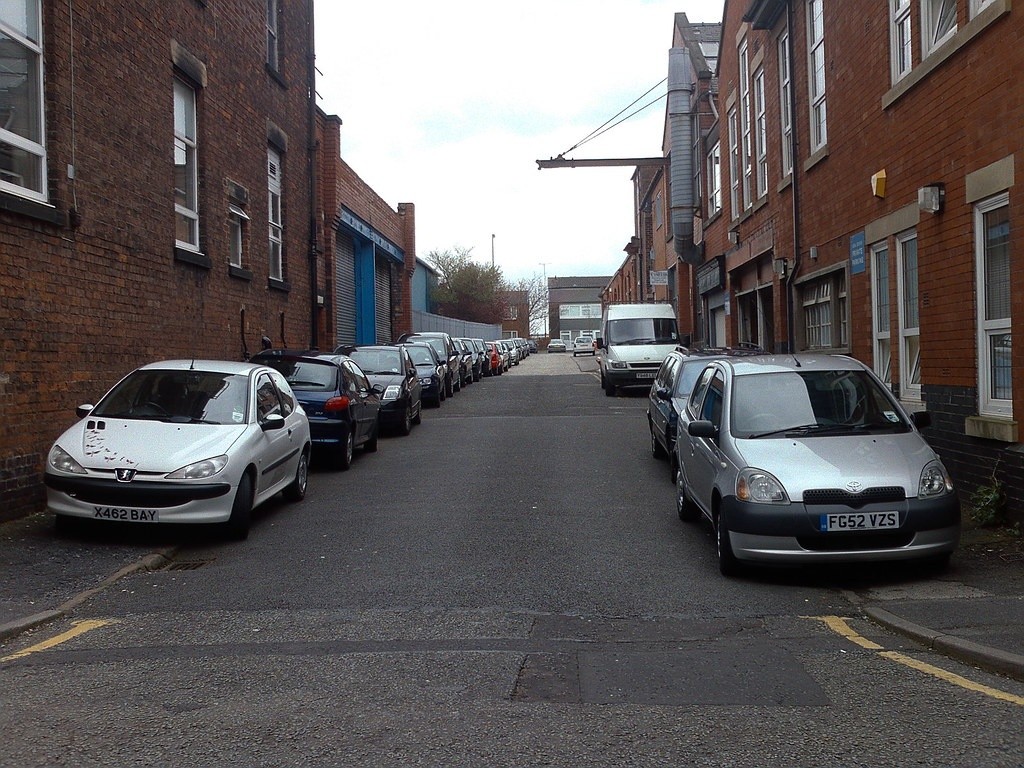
[647,339,775,482]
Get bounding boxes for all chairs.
[150,375,187,414]
[414,352,430,363]
[178,390,210,420]
[381,356,401,371]
[352,355,366,369]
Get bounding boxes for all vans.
[595,299,692,398]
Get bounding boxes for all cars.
[546,339,567,353]
[386,332,539,409]
[571,335,596,357]
[328,343,425,436]
[41,359,312,540]
[249,347,385,471]
[672,351,962,576]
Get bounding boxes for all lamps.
[773,257,787,275]
[727,231,740,246]
[918,181,946,218]
[609,287,613,293]
